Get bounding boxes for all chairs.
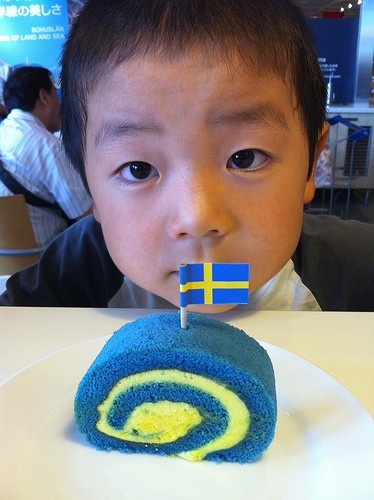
[0,195,44,278]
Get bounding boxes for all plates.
[0,337,374,500]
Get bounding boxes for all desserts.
[72,312,279,464]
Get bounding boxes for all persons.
[0,0,374,314]
[0,64,93,256]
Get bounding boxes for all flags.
[180,262,250,306]
